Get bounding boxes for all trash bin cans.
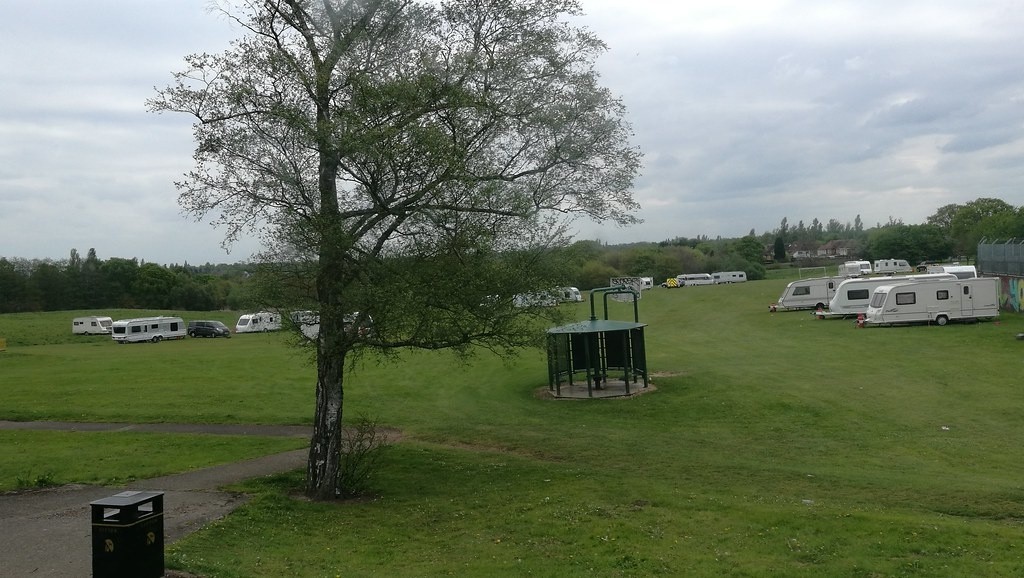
[89,490,165,578]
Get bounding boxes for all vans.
[186,320,231,338]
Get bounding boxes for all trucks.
[71,314,187,344]
[711,270,747,285]
[236,308,374,337]
[479,285,586,309]
[641,276,652,290]
[677,273,714,287]
[767,257,1002,329]
